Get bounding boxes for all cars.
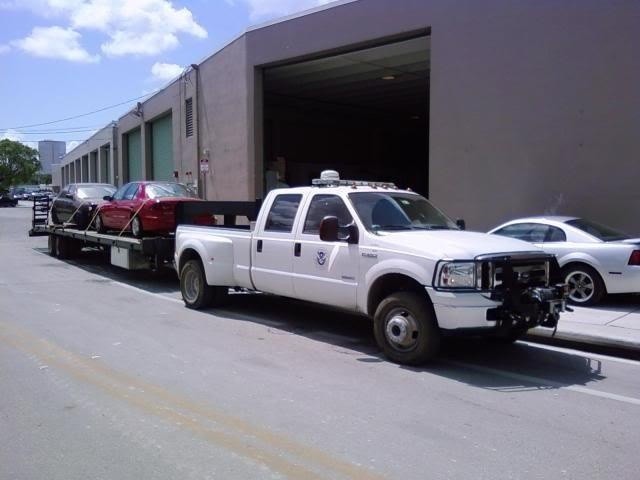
[485,216,640,307]
[0,183,56,207]
[50,182,119,231]
[94,180,218,239]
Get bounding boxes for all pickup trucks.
[172,176,574,368]
